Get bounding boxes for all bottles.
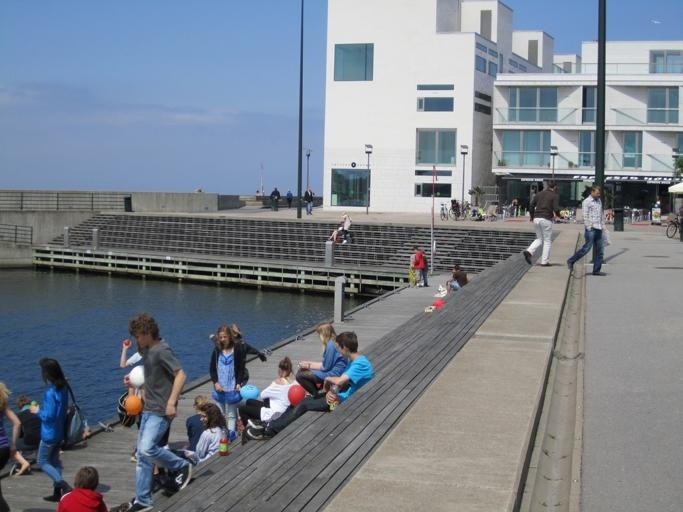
[219,430,229,456]
[330,388,339,412]
[30,401,38,410]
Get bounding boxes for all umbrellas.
[668,182,682,195]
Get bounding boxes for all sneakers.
[174,462,192,490]
[121,498,154,512]
[592,271,609,276]
[523,251,532,264]
[247,428,273,441]
[542,263,552,266]
[566,260,574,274]
[248,418,268,431]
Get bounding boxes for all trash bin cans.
[124,197,131,212]
[614,208,624,231]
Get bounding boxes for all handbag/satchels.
[65,383,85,448]
[118,390,135,428]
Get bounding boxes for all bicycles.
[664,216,680,237]
[440,200,466,222]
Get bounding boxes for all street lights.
[363,145,373,215]
[549,146,558,187]
[305,145,312,189]
[459,144,468,219]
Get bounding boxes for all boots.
[56,481,72,494]
[42,487,61,503]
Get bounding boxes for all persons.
[121,323,375,494]
[127,315,192,511]
[410,245,425,287]
[0,357,110,512]
[328,222,344,243]
[422,252,428,286]
[446,264,467,293]
[285,189,292,207]
[303,188,315,215]
[341,211,352,245]
[521,180,564,266]
[566,184,605,276]
[269,187,281,211]
